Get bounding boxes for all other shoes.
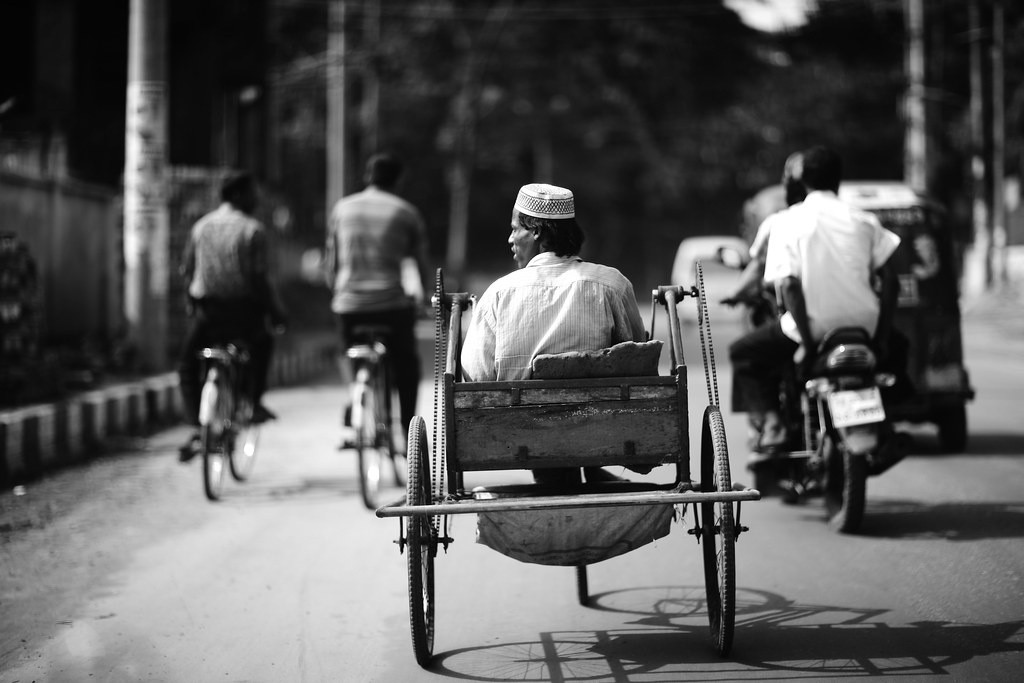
[749,436,777,455]
[248,408,276,422]
[179,434,200,462]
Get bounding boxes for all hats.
[514,182,575,220]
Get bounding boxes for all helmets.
[783,152,808,190]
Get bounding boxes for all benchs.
[435,364,690,491]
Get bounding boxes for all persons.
[176,172,276,464]
[460,182,648,496]
[728,144,901,453]
[323,152,438,458]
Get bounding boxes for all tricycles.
[372,260,761,672]
[674,180,979,456]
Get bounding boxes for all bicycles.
[337,287,435,512]
[187,272,276,502]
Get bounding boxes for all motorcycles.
[715,275,899,536]
